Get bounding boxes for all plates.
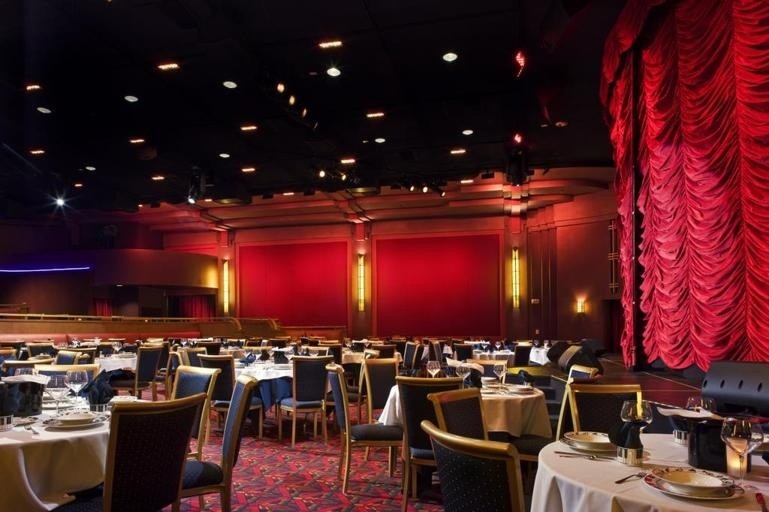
[515,385,531,390]
[512,391,534,394]
[59,413,96,423]
[481,390,494,393]
[653,467,734,494]
[561,439,616,452]
[564,431,613,448]
[645,474,745,501]
[43,411,111,428]
[751,432,769,451]
[43,403,74,409]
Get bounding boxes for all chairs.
[396,376,466,512]
[454,342,473,361]
[26,343,57,357]
[400,342,420,370]
[168,365,221,509]
[197,353,263,440]
[109,347,162,401]
[227,338,246,347]
[346,348,380,423]
[512,345,533,366]
[0,349,17,377]
[326,363,404,492]
[467,358,508,383]
[102,392,207,512]
[516,364,598,462]
[198,343,222,355]
[373,345,397,358]
[399,344,424,377]
[55,350,82,364]
[428,388,489,442]
[270,339,288,348]
[187,347,207,367]
[429,340,449,376]
[144,342,176,405]
[3,359,54,376]
[566,383,644,432]
[300,346,330,356]
[318,343,343,364]
[421,419,526,512]
[277,355,336,448]
[183,374,258,511]
[35,364,99,388]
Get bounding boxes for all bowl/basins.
[657,406,712,432]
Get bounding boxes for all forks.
[615,468,648,484]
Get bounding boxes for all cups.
[426,360,441,378]
[686,396,717,418]
[622,399,652,452]
[494,361,507,393]
[115,342,122,354]
[482,342,488,351]
[534,340,539,347]
[721,415,764,493]
[456,364,470,384]
[181,338,187,347]
[64,368,89,400]
[495,341,501,350]
[45,375,70,415]
[72,338,78,346]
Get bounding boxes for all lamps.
[357,253,366,312]
[512,246,521,309]
[224,260,229,315]
[576,299,586,313]
